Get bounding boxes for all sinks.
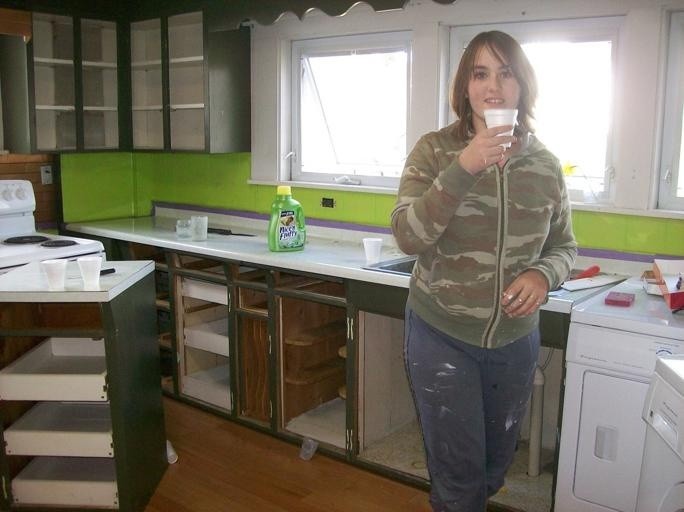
[377,256,593,299]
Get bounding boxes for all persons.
[388,29,579,512]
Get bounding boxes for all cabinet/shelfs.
[0,259,169,512]
[92,236,351,462]
[0,1,250,154]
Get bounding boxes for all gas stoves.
[0,231,105,269]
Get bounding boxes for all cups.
[176,216,209,241]
[166,439,179,465]
[40,258,68,291]
[77,255,103,289]
[362,236,383,266]
[482,109,519,149]
[299,438,319,461]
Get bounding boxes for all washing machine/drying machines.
[552,275,684,512]
[635,356,684,511]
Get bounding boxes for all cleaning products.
[268,185,306,252]
